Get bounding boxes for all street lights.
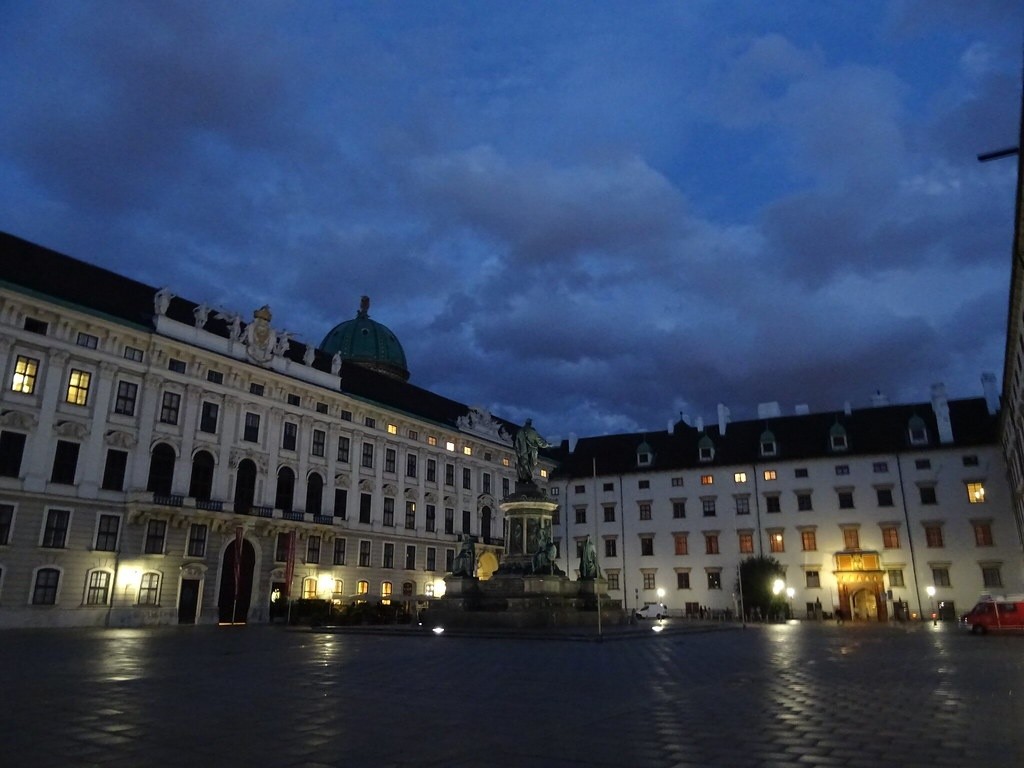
[659,589,664,622]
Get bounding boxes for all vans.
[635,605,669,620]
[958,595,1024,634]
